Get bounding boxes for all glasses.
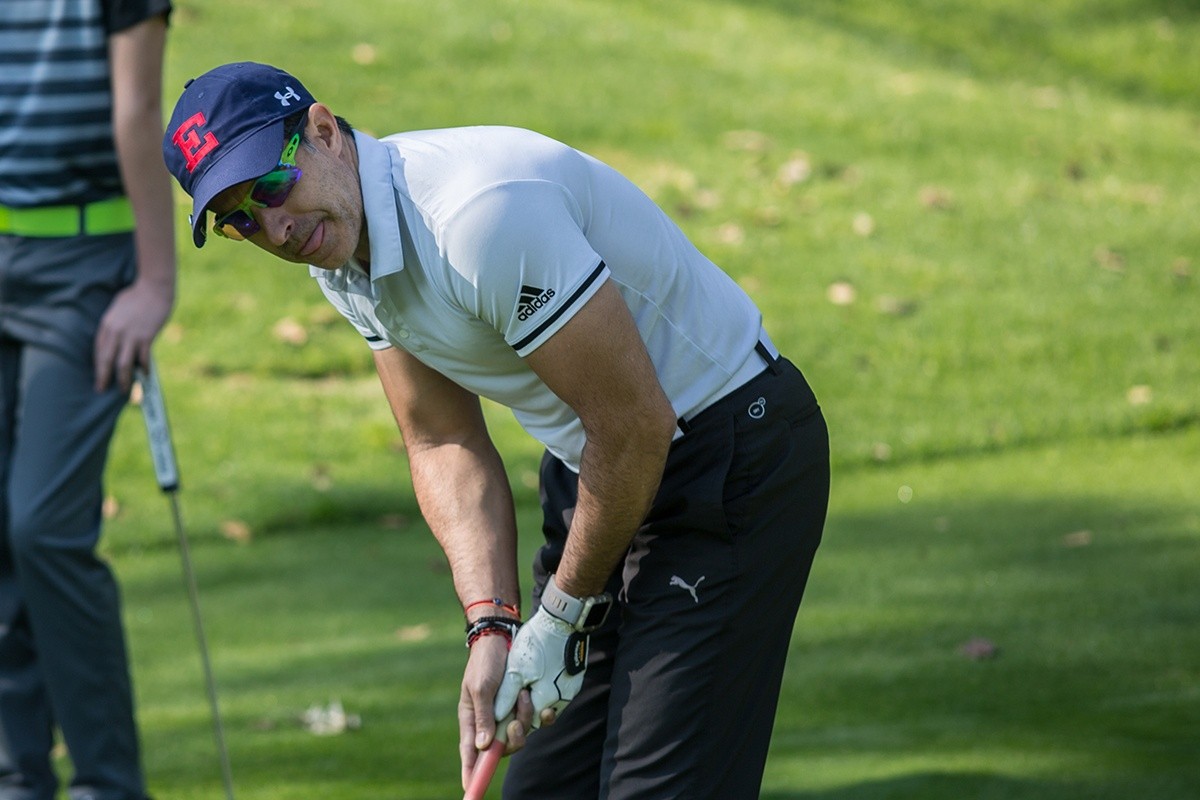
[213,107,308,241]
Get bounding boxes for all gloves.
[494,605,589,737]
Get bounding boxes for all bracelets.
[462,598,524,651]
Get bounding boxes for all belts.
[673,329,779,441]
[0,195,134,237]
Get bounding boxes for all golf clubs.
[462,708,515,800]
[132,351,234,799]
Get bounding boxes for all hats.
[164,62,317,248]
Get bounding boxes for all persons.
[159,65,833,800]
[0,0,175,800]
